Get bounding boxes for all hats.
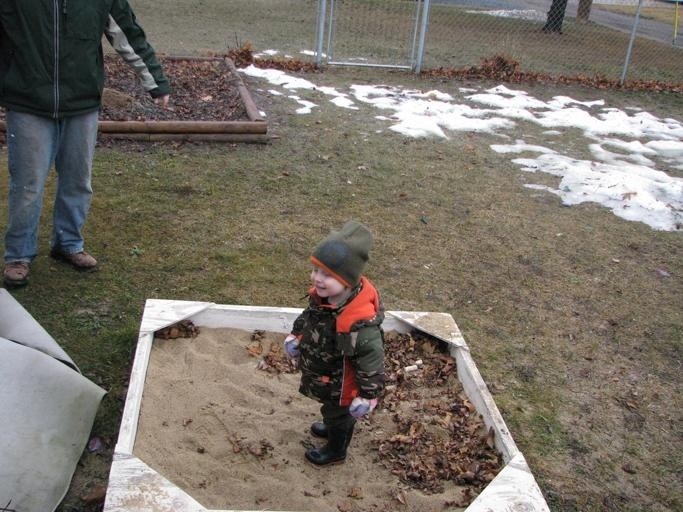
[308,218,375,290]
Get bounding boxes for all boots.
[305,421,356,466]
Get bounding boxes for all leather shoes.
[3,259,32,286]
[50,247,98,272]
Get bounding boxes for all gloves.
[349,396,378,419]
[282,334,300,360]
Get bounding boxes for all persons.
[284,220,389,466]
[0,0,169,289]
